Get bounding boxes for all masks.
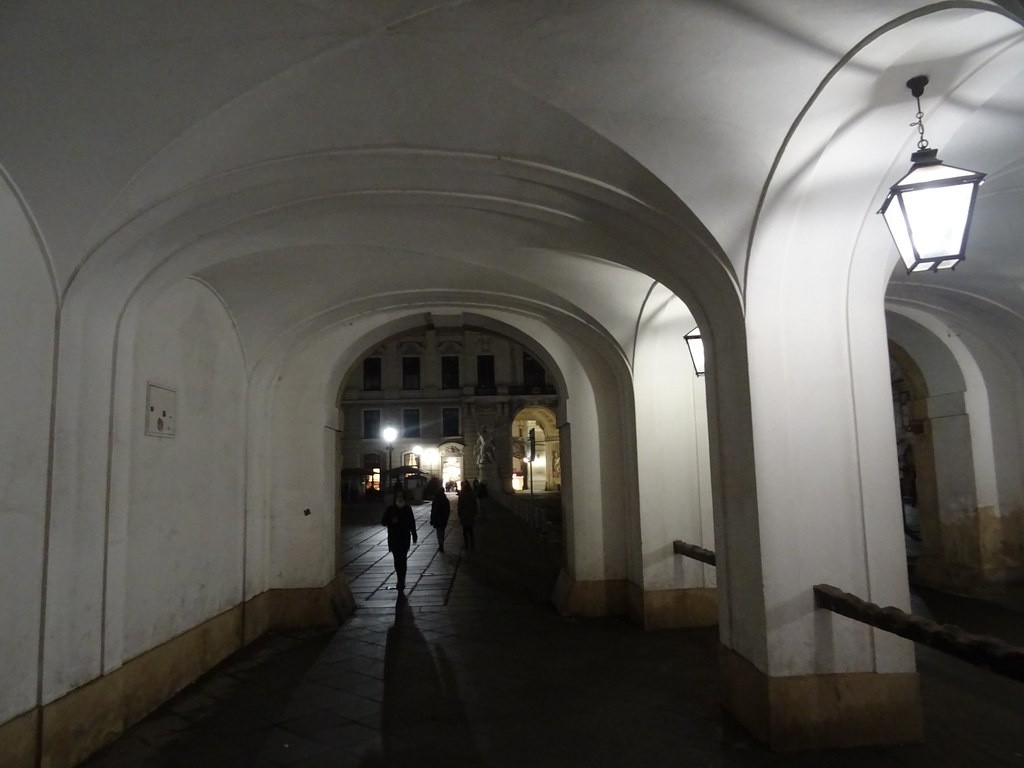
[396,501,405,508]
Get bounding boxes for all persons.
[381,490,418,592]
[457,478,487,548]
[446,480,459,492]
[429,486,452,552]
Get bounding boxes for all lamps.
[876,75,992,275]
[685,325,706,377]
